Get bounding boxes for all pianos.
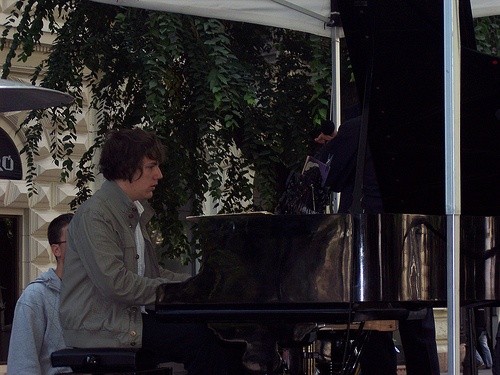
[143,215,496,370]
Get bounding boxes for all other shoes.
[477,363,486,369]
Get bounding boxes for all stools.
[50,350,172,375]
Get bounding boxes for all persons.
[315,114,440,375]
[7,213,74,375]
[59,129,226,375]
